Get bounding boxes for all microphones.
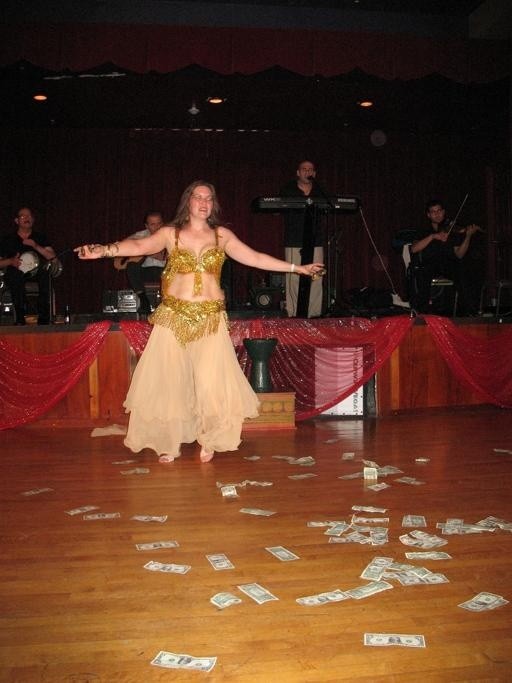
[306,176,327,184]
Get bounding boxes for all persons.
[268,157,333,319]
[2,204,60,327]
[407,199,486,318]
[110,209,169,316]
[72,180,327,465]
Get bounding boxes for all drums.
[18,250,63,280]
[243,337,278,393]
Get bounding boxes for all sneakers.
[14,320,50,325]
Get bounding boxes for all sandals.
[200,446,215,463]
[158,454,175,464]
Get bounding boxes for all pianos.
[249,194,359,215]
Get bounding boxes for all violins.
[441,219,489,237]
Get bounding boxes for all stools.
[430,279,460,318]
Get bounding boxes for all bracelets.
[102,241,121,258]
[430,232,435,240]
[290,263,295,272]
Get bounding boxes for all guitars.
[113,237,147,271]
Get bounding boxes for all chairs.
[479,240,512,319]
